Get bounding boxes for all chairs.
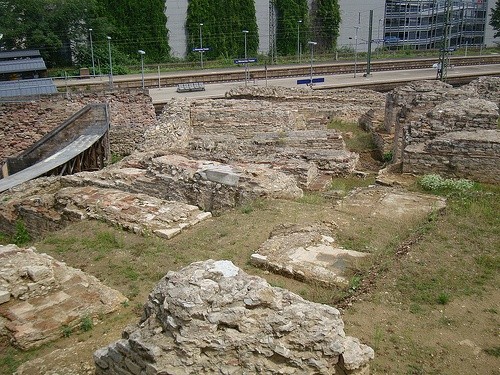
[177,83,205,92]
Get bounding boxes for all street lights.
[378,18,383,41]
[89,29,96,78]
[242,30,249,88]
[354,26,360,78]
[138,50,146,90]
[308,42,317,85]
[107,37,113,86]
[297,20,302,62]
[199,23,204,68]
